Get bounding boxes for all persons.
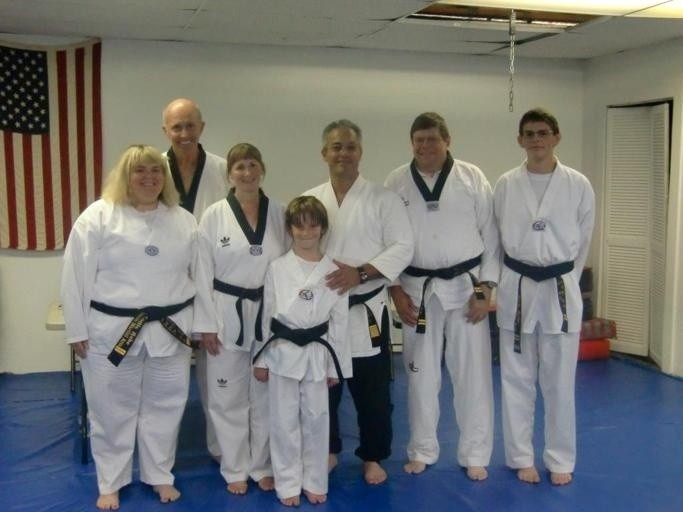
[301,115,413,486]
[252,194,352,507]
[156,96,229,222]
[383,110,500,483]
[199,139,289,494]
[61,142,201,512]
[492,110,597,486]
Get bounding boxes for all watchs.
[356,266,369,285]
[481,281,499,289]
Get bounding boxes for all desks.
[42,301,86,397]
